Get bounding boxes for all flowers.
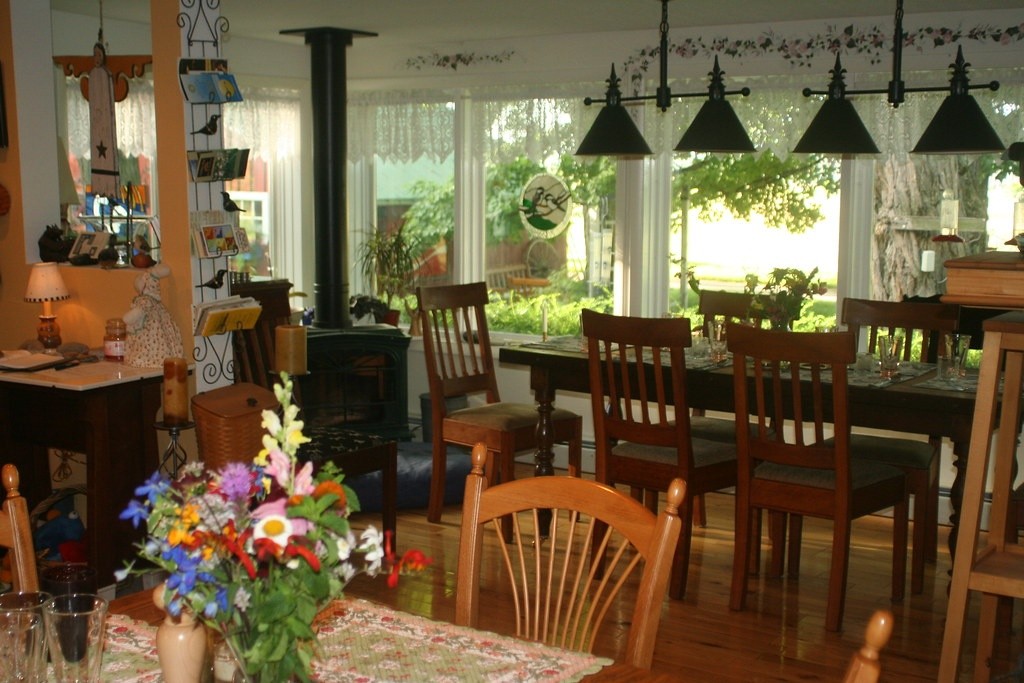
[112,371,375,630]
[739,271,822,320]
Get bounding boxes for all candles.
[161,355,188,429]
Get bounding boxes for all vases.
[765,311,793,370]
[210,617,299,683]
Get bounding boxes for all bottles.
[103,319,126,362]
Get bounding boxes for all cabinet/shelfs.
[939,310,1024,683]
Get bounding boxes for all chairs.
[417,281,582,542]
[578,310,784,601]
[451,438,686,683]
[724,320,908,632]
[0,463,42,596]
[231,309,397,554]
[790,294,965,604]
[644,291,807,530]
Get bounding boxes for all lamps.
[794,0,1006,155]
[931,182,965,242]
[575,0,757,156]
[23,261,70,348]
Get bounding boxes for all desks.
[0,350,191,601]
[0,569,684,683]
[499,336,1024,594]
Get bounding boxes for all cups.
[688,336,709,359]
[854,352,876,379]
[878,335,904,378]
[0,612,41,682]
[814,326,840,334]
[41,594,108,683]
[580,314,588,352]
[945,334,972,378]
[0,591,51,683]
[936,355,959,382]
[706,320,729,362]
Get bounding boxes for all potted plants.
[356,221,420,325]
